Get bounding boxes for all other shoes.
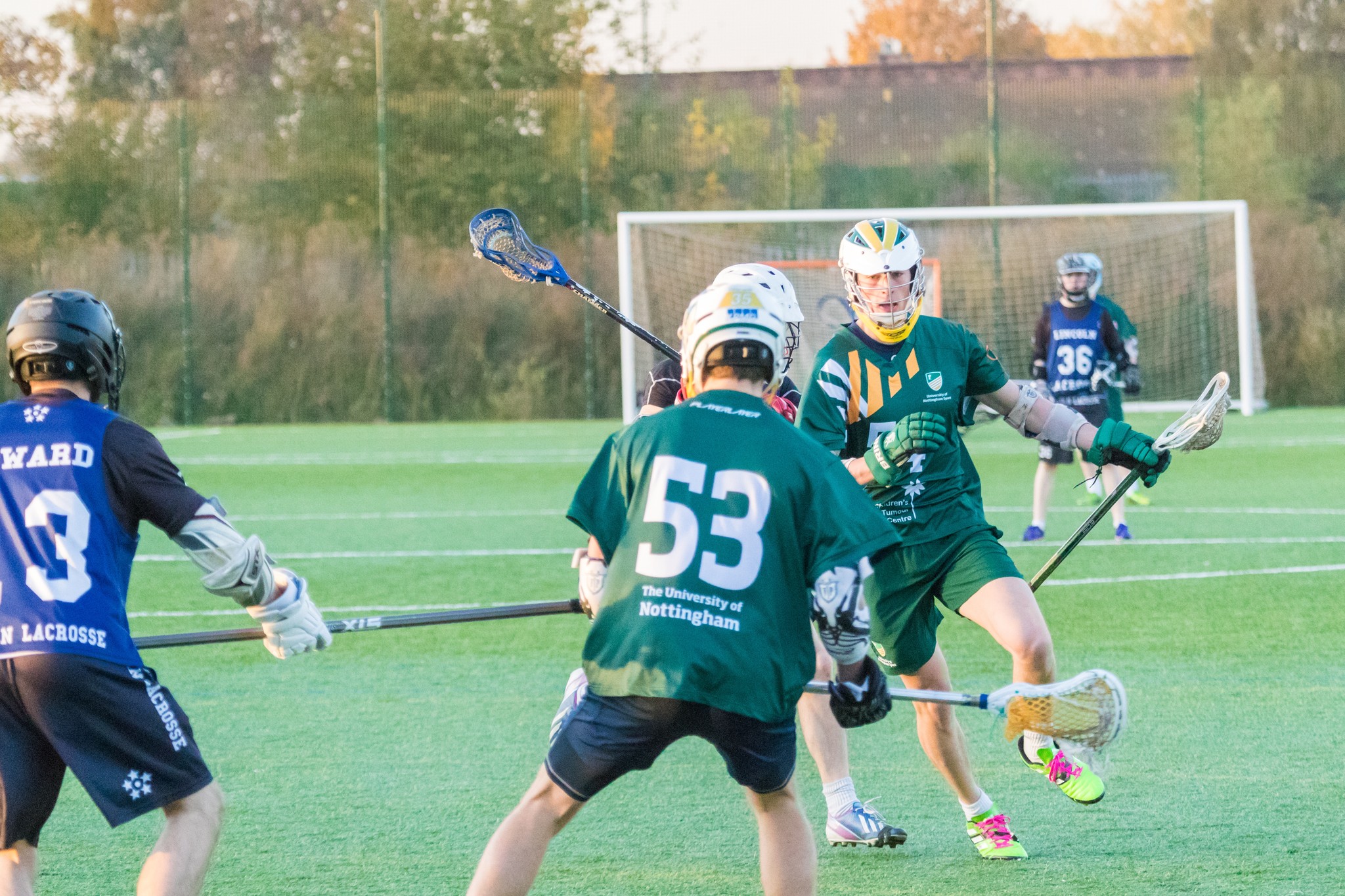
[1024,526,1043,542]
[1115,524,1132,539]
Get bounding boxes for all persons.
[466,218,1172,896]
[0,289,332,896]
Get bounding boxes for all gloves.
[246,568,331,660]
[1074,417,1171,487]
[862,411,947,486]
[824,658,893,728]
[1123,364,1142,394]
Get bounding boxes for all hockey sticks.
[803,669,1127,753]
[128,598,595,650]
[469,207,682,365]
[968,380,1127,432]
[1028,370,1231,593]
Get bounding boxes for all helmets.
[1080,254,1102,299]
[1058,254,1090,276]
[688,283,786,394]
[713,263,804,331]
[839,217,919,311]
[6,290,123,392]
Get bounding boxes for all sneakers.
[1017,735,1107,805]
[826,794,908,850]
[965,806,1029,859]
